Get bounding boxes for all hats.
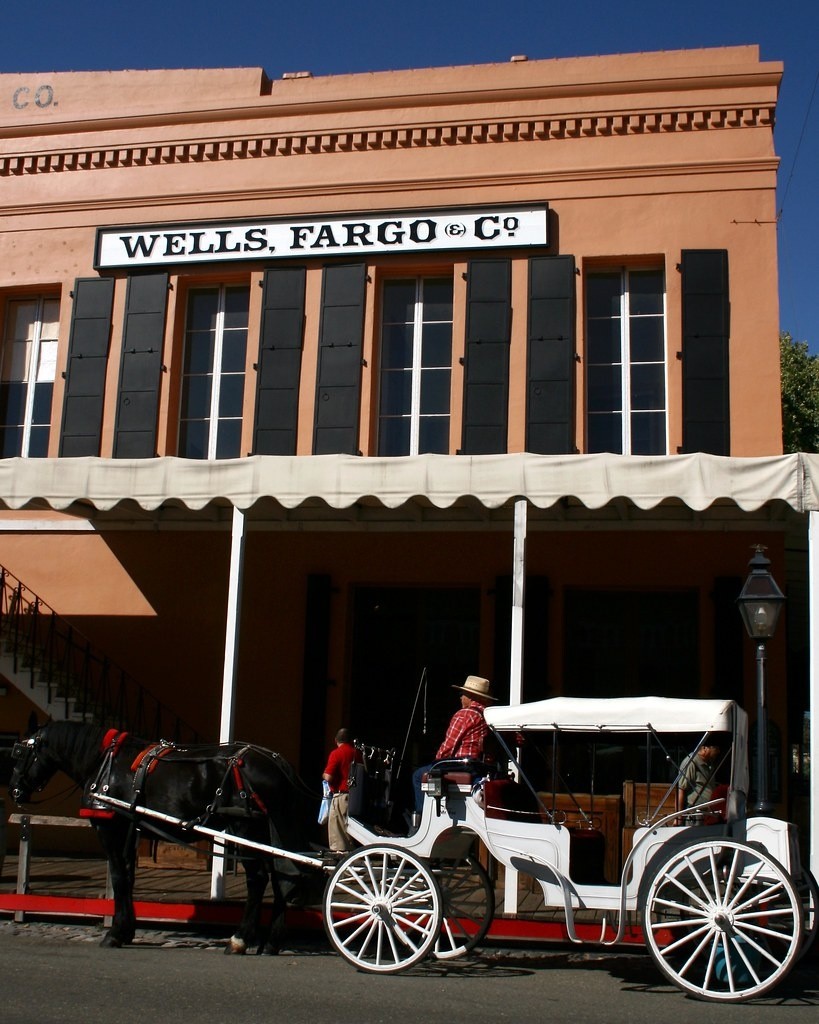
[450,675,498,702]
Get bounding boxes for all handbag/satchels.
[318,780,334,825]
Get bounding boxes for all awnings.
[0,452,819,514]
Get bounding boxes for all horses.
[8,719,305,957]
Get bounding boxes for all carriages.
[6,698,819,1005]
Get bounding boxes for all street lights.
[733,545,788,824]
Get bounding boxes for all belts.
[334,791,349,793]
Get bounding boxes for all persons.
[323,727,367,891]
[412,675,498,836]
[672,732,722,826]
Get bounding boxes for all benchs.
[414,760,604,882]
[631,785,747,878]
